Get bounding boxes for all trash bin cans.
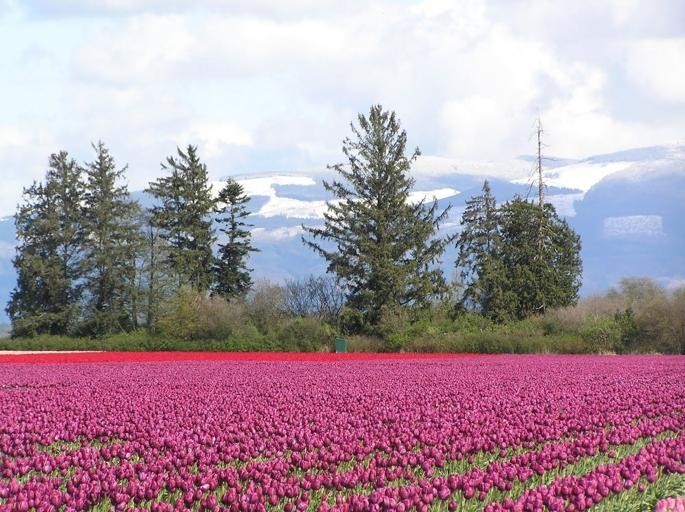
[335,339,348,354]
